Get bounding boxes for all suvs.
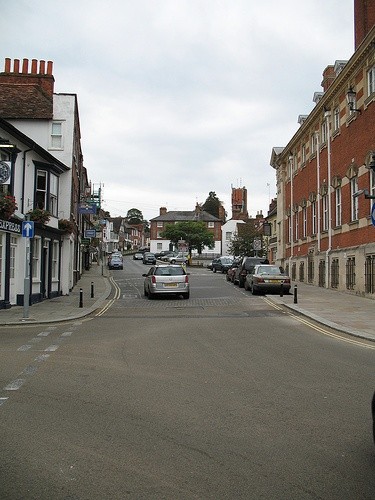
[233,257,272,289]
[142,253,157,264]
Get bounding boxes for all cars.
[244,264,291,295]
[210,256,236,275]
[132,253,143,260]
[142,264,191,300]
[108,249,124,270]
[154,250,188,263]
[226,256,242,282]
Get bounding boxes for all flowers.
[30,208,50,226]
[0,193,18,221]
[58,218,75,232]
